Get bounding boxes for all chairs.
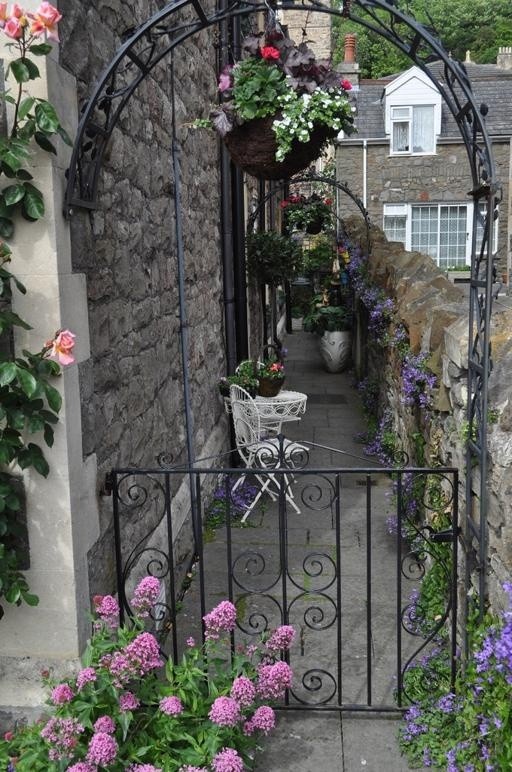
[218,360,308,523]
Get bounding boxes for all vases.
[299,220,322,234]
[221,110,326,183]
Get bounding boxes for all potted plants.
[318,306,355,374]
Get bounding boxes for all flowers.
[178,29,361,164]
[281,187,333,232]
